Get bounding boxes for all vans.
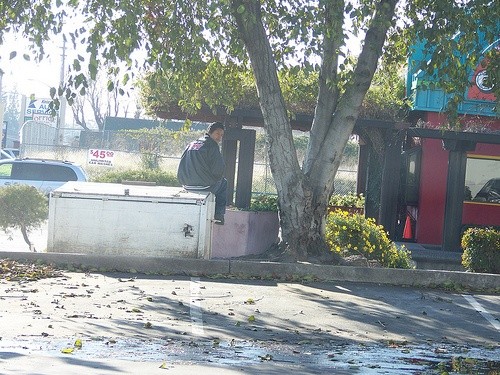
[470,177,500,239]
[0,156,89,207]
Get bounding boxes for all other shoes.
[215,214,224,224]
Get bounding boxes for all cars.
[1,148,19,164]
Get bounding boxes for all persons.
[177,121,228,226]
